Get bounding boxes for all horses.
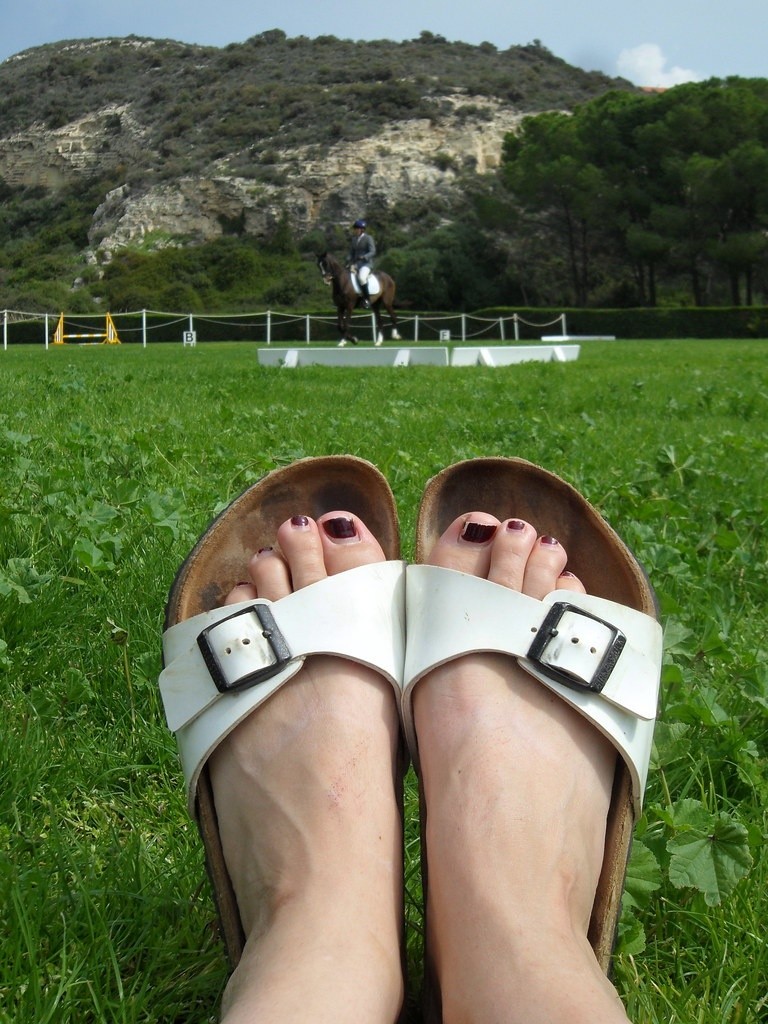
[312,248,407,349]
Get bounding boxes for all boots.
[361,283,371,309]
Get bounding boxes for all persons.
[344,220,376,309]
[156,456,664,1024]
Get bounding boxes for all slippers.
[159,453,658,1024]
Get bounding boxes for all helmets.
[353,220,367,229]
